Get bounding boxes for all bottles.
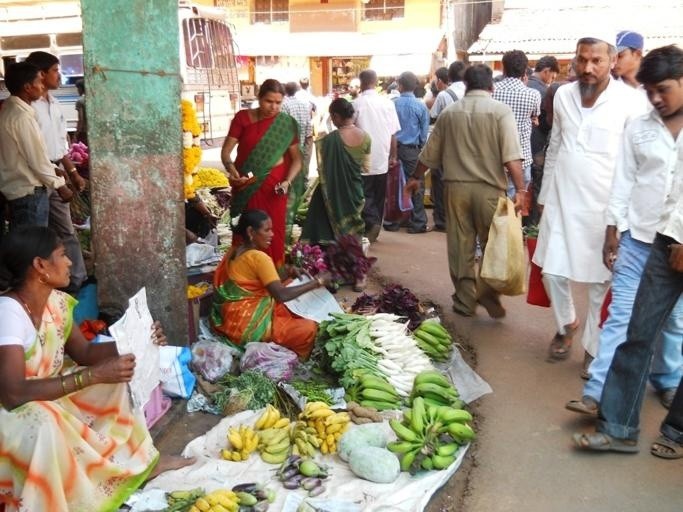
[616,32,643,53]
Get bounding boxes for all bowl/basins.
[256,107,265,134]
[12,287,49,345]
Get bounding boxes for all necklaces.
[12,287,49,345]
[256,107,265,134]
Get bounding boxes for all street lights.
[383,159,414,222]
[526,238,549,307]
[479,197,529,295]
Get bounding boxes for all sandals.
[0,5,241,155]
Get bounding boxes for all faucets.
[649,374,676,408]
[581,350,593,379]
[565,395,600,415]
[651,435,683,459]
[549,316,580,361]
[572,431,638,453]
[365,225,380,243]
[408,224,432,233]
[476,291,504,318]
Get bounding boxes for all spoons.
[80,319,106,341]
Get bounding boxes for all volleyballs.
[276,455,328,497]
[232,484,274,512]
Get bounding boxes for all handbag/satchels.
[72,373,77,392]
[67,167,77,176]
[77,370,83,390]
[86,365,91,386]
[224,160,233,169]
[60,376,69,397]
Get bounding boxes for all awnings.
[463,22,681,62]
[231,22,446,58]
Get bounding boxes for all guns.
[414,319,452,361]
[221,368,475,472]
[188,491,240,512]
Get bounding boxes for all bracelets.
[60,376,69,397]
[317,278,325,286]
[411,173,423,180]
[72,373,77,392]
[515,189,528,193]
[224,160,233,169]
[77,370,83,390]
[67,167,77,176]
[86,365,91,386]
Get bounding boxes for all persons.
[277,31,681,459]
[0,222,197,511]
[220,78,302,266]
[0,61,74,231]
[209,209,331,364]
[24,51,87,293]
[71,79,86,148]
